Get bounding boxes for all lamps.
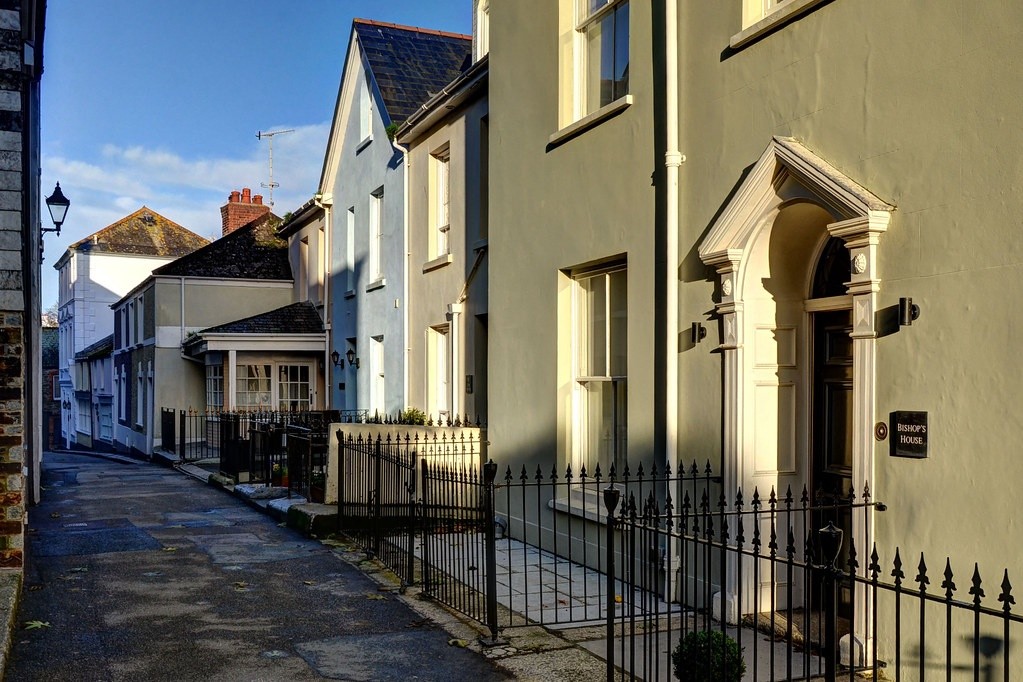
[330,349,344,369]
[40,180,71,237]
[346,348,360,369]
[898,297,920,326]
[691,322,707,344]
